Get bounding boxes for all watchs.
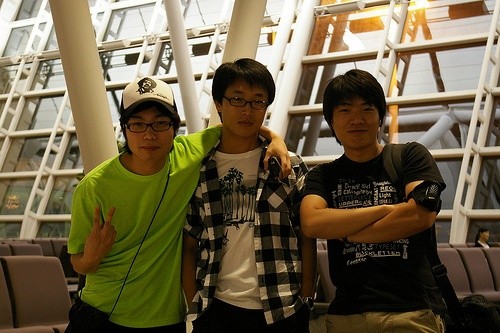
[300,296,315,312]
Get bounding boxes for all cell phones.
[269,157,280,178]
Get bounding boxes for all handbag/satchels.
[64,301,108,333]
[452,295,500,333]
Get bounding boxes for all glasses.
[223,97,269,110]
[125,120,173,133]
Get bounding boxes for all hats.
[120,77,176,114]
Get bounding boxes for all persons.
[63,77,294,333]
[178,58,317,333]
[297,69,445,333]
[475,227,490,248]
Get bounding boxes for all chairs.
[436,242,500,315]
[0,237,76,333]
[316,238,336,300]
